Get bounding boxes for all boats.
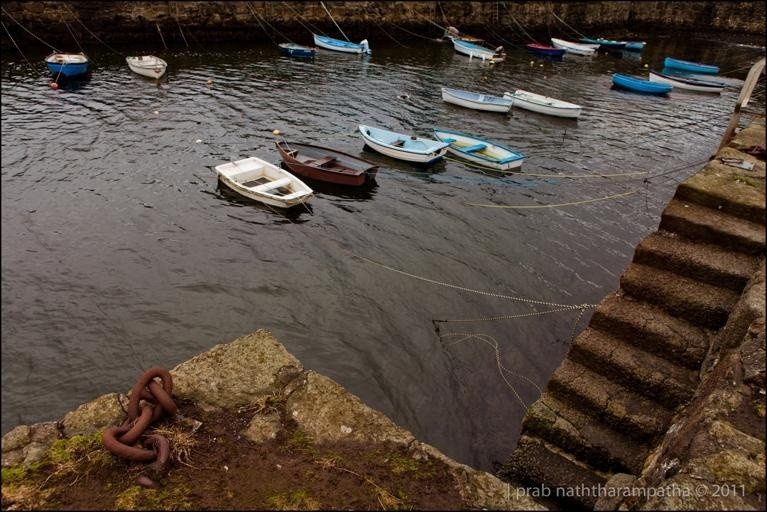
[664,58,721,74]
[451,38,507,63]
[442,87,514,112]
[551,38,601,56]
[444,27,485,44]
[46,51,88,79]
[215,157,313,208]
[433,128,526,170]
[649,71,725,93]
[613,74,673,93]
[579,38,647,50]
[503,90,582,118]
[359,123,451,163]
[279,43,315,59]
[126,55,167,79]
[526,43,568,62]
[313,33,369,54]
[276,140,379,185]
